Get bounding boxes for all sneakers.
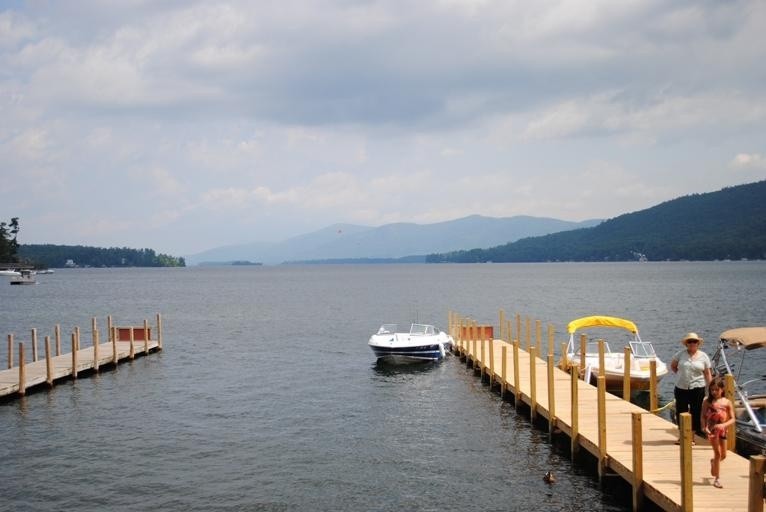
[714,480,723,489]
[710,458,718,477]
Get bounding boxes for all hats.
[682,332,703,346]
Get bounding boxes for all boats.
[368,324,453,366]
[0,269,54,285]
[567,315,669,392]
[670,327,766,456]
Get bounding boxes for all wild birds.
[544,469,554,483]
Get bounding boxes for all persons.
[700,377,737,489]
[671,333,713,446]
